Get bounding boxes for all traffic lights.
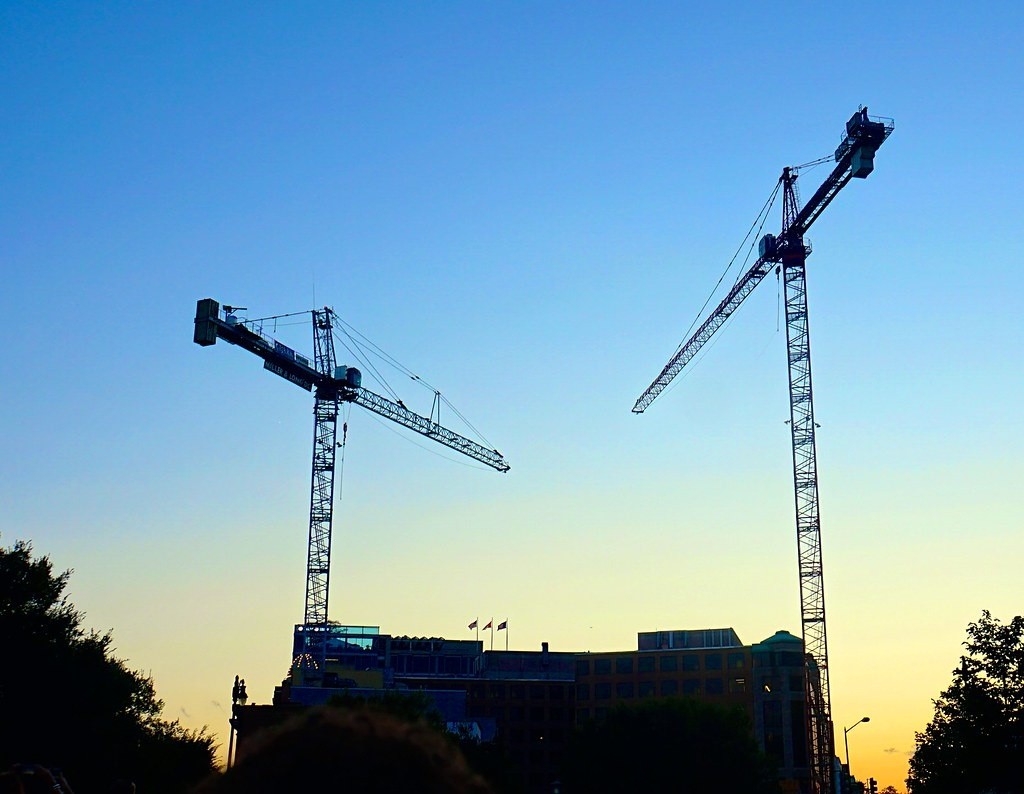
[869,777,878,794]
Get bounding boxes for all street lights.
[227,675,249,769]
[844,718,870,765]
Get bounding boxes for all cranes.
[192,282,511,631]
[630,102,895,793]
